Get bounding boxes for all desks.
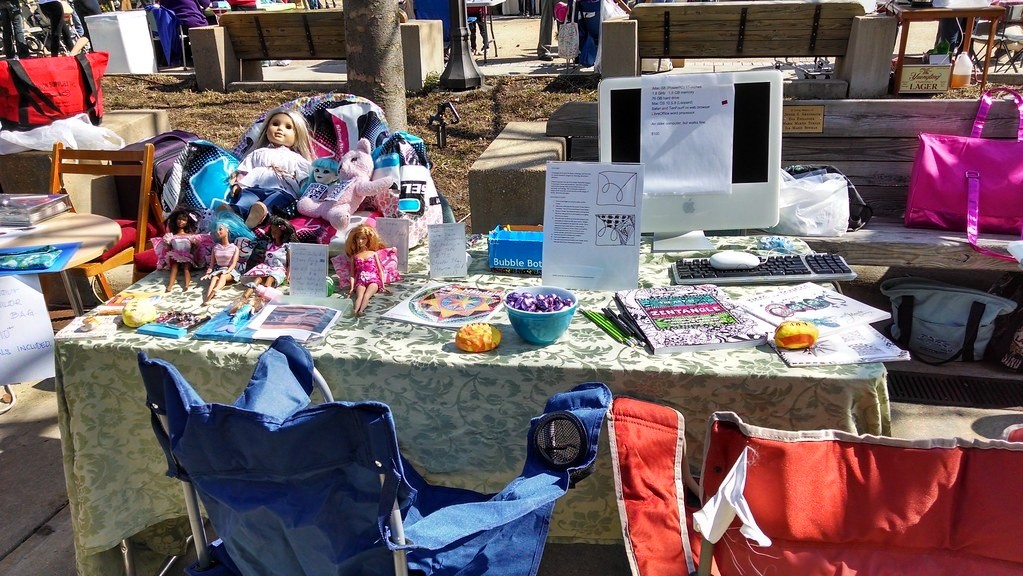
[878,1,1007,90]
[53,234,893,576]
[1,211,122,319]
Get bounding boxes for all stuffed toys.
[297,138,393,231]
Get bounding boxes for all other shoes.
[539,55,554,61]
[471,48,490,56]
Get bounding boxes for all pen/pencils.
[578,296,658,355]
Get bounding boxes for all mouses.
[709,251,760,269]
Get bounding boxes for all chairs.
[138,336,612,576]
[607,397,1023,576]
[969,4,1023,73]
[46,140,155,314]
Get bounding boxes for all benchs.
[469,99,1023,288]
[601,0,895,98]
[191,7,443,93]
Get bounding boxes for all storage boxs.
[899,54,952,94]
[83,8,158,75]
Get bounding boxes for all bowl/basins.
[502,285,579,345]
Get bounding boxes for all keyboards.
[671,253,857,285]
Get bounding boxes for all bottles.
[952,52,973,88]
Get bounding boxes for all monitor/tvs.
[599,69,783,252]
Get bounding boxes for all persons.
[200,210,240,305]
[38,0,74,57]
[150,206,212,292]
[229,219,295,314]
[217,106,314,230]
[537,0,566,61]
[941,16,980,60]
[160,0,210,68]
[0,0,31,60]
[153,0,160,8]
[70,0,102,55]
[345,224,390,316]
[227,0,257,11]
[467,6,490,56]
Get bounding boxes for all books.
[0,194,72,227]
[616,284,768,355]
[732,281,911,367]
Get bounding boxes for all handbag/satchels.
[0,0,110,129]
[904,86,1022,237]
[878,275,1016,366]
[558,23,579,58]
